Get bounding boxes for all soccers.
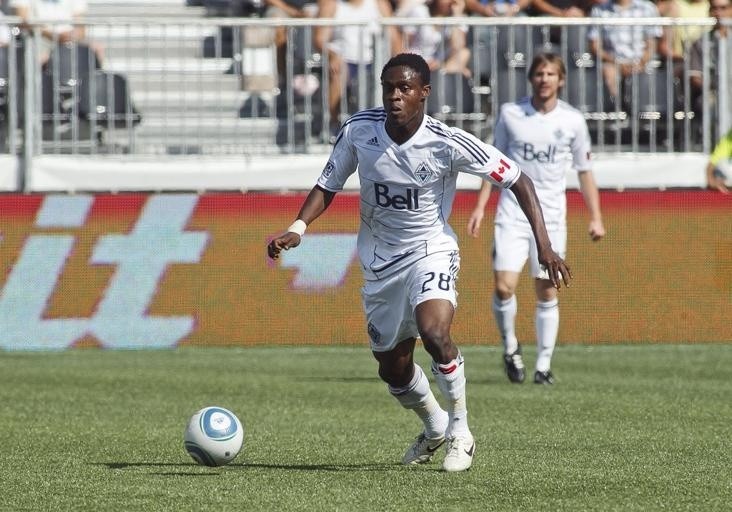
[184,407,244,464]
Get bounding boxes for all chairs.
[329,20,699,147]
[1,39,141,154]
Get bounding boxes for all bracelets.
[287,219,307,237]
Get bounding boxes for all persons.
[269,51,575,474]
[222,2,730,148]
[465,54,607,383]
[8,1,106,71]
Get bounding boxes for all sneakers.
[502,343,528,384]
[533,368,554,385]
[442,434,476,473]
[400,426,448,464]
